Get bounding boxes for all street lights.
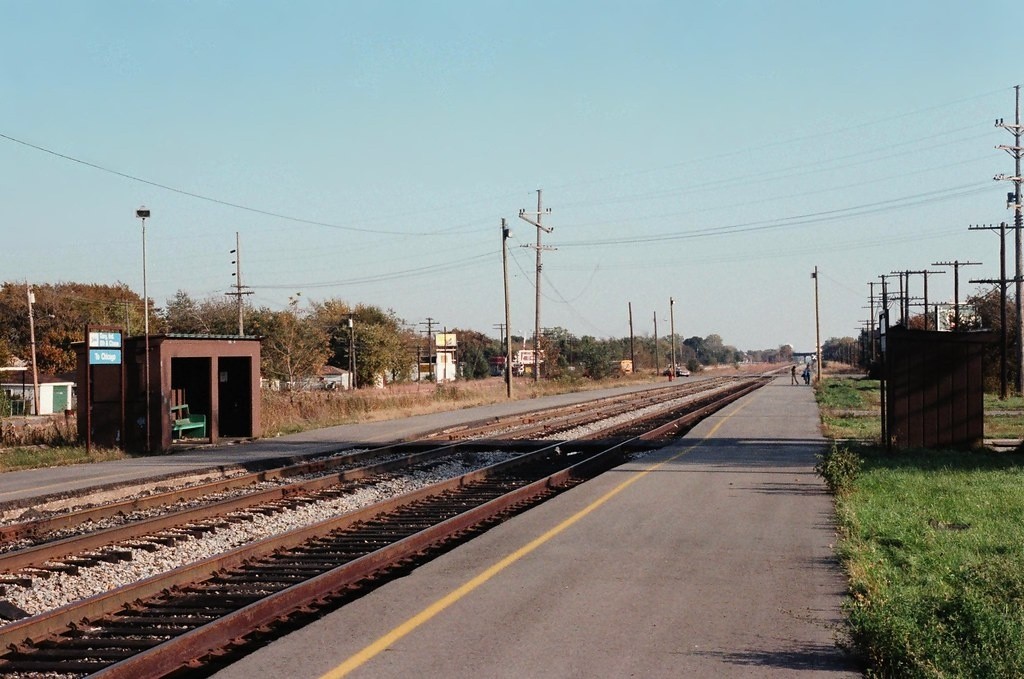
[29,314,55,413]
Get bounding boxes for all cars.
[662,363,690,377]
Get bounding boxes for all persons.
[791,365,799,386]
[669,365,673,382]
[804,364,811,385]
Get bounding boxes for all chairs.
[170,404,205,440]
